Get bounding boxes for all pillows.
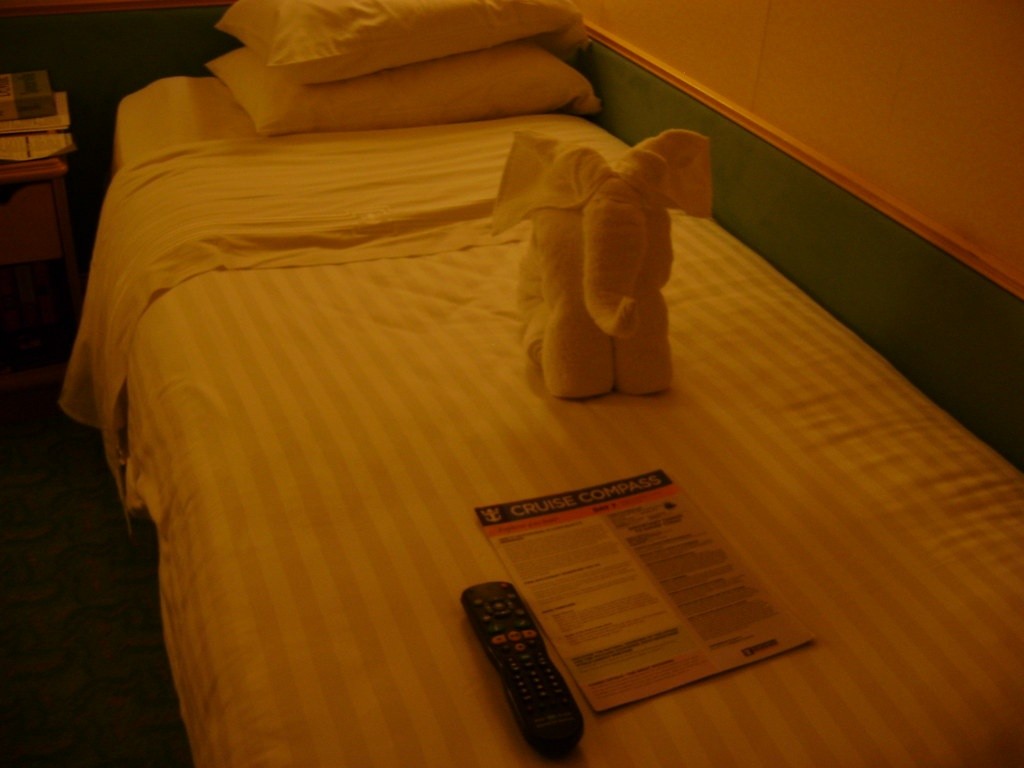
[204,45,602,137]
[215,0,595,87]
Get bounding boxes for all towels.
[487,123,715,400]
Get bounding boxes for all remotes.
[460,581,584,762]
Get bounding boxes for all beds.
[57,77,1024,768]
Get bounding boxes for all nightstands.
[0,154,85,396]
